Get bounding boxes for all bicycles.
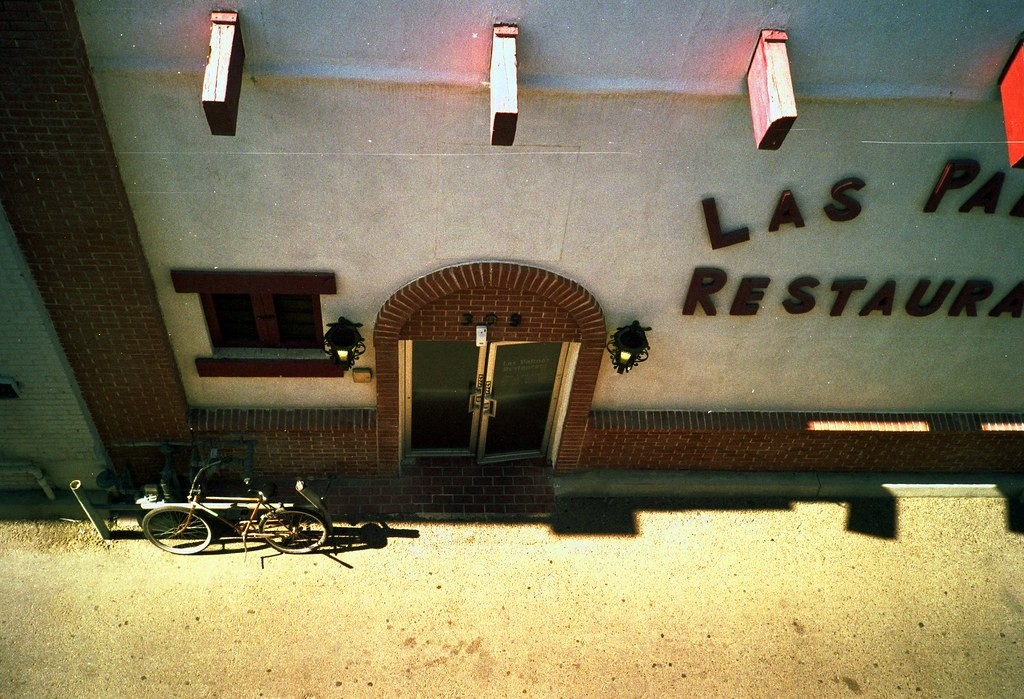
[141,427,335,556]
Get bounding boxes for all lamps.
[607,320,652,376]
[319,316,367,374]
